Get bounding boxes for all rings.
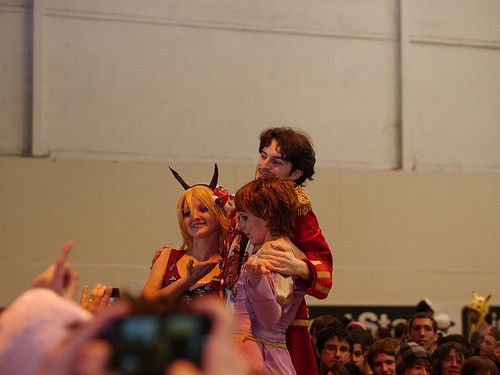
[87,297,95,304]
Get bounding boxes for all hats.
[347,321,366,331]
[461,290,491,342]
[0,287,95,375]
[394,342,430,375]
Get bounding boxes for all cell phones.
[104,288,120,307]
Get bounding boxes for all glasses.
[323,344,350,355]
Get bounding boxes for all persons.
[0,237,263,375]
[234,181,309,375]
[309,303,500,375]
[140,164,234,310]
[229,124,333,375]
